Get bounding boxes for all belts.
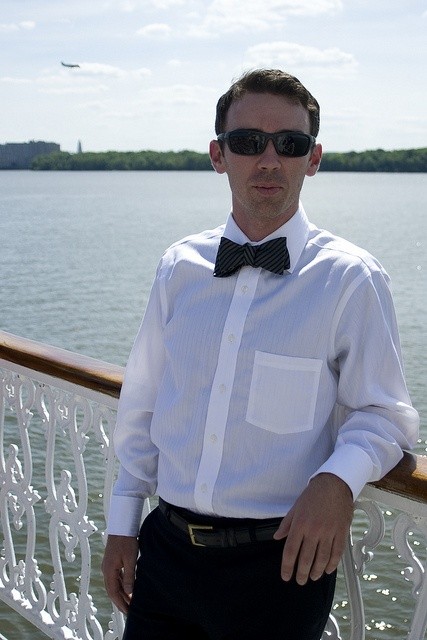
[156,496,288,549]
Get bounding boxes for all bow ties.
[212,236,291,278]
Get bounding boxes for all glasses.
[216,127,317,158]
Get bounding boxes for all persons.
[102,69,421,637]
